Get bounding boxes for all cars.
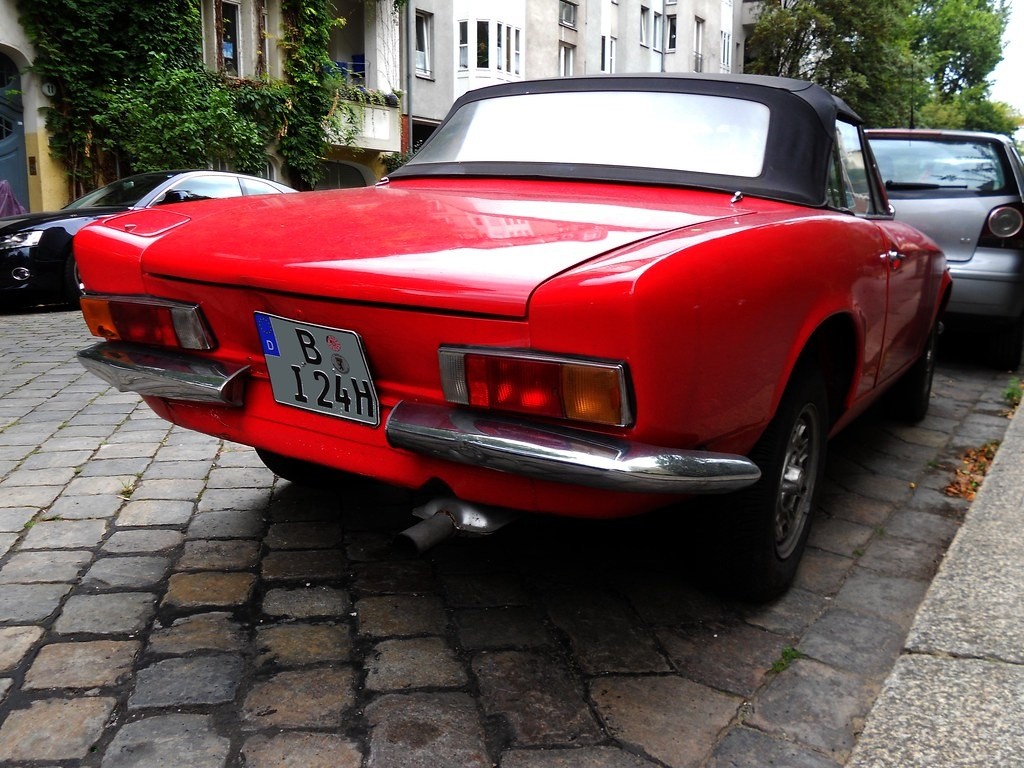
[1,168,305,319]
[855,130,1024,353]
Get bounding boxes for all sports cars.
[69,72,950,609]
